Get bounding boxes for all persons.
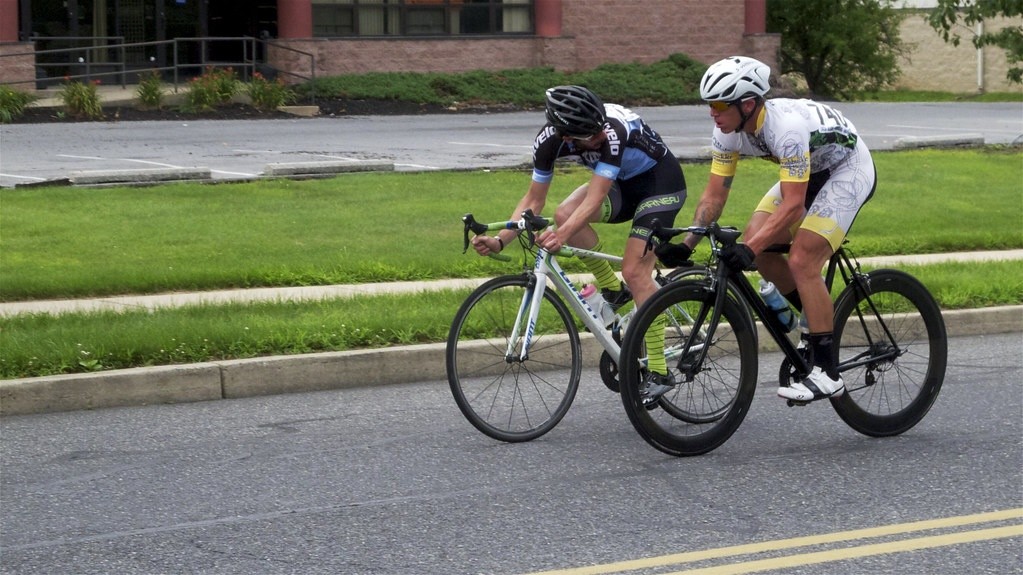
[653,56,878,401]
[470,85,687,406]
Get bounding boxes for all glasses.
[706,99,737,113]
[570,130,604,142]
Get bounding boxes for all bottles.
[759,279,798,331]
[799,275,825,334]
[579,284,615,328]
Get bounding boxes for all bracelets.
[494,236,504,251]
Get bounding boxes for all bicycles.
[445,208,762,443]
[618,217,948,457]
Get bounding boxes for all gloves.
[721,243,759,272]
[654,241,693,269]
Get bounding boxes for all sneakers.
[788,318,811,374]
[585,279,634,333]
[777,365,844,401]
[639,367,676,407]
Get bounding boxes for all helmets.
[699,55,771,102]
[545,84,607,136]
